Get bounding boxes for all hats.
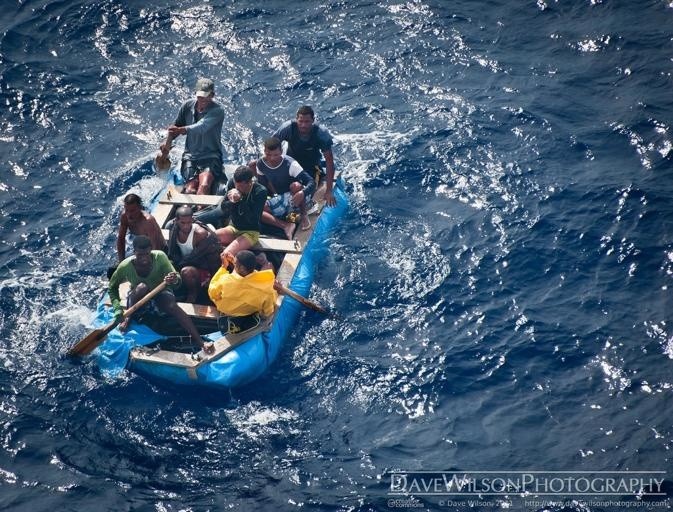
[196,78,215,98]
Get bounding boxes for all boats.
[94,164,350,395]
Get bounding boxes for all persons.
[165,206,221,303]
[115,193,169,258]
[211,165,268,263]
[105,235,214,351]
[270,105,336,209]
[158,77,225,209]
[205,248,282,317]
[254,136,317,240]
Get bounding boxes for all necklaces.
[237,184,252,216]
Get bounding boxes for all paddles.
[68,272,173,359]
[223,252,332,315]
[154,129,175,172]
